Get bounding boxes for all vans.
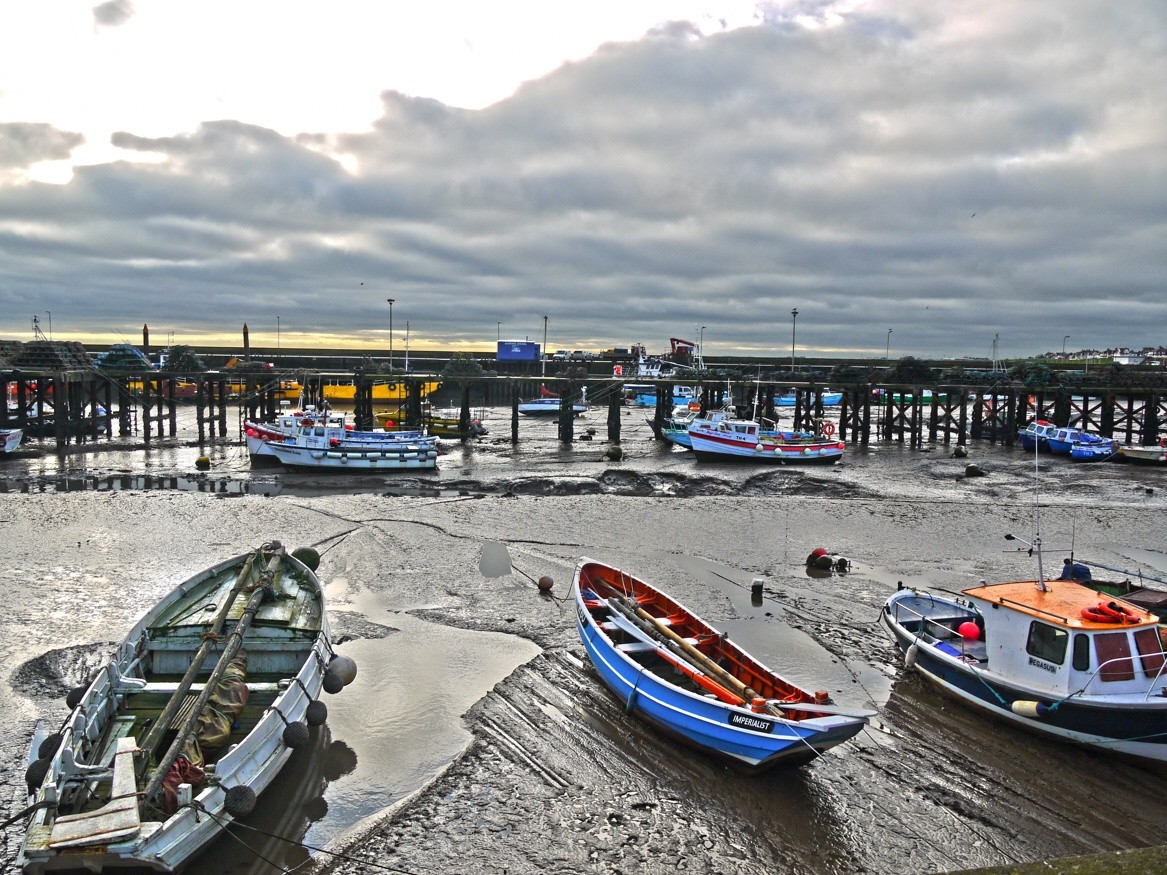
[553,349,572,362]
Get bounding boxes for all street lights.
[542,314,549,396]
[791,307,799,377]
[387,296,396,375]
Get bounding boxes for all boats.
[1,427,24,454]
[573,555,881,776]
[1048,427,1101,456]
[1071,434,1121,460]
[24,540,358,874]
[129,355,444,401]
[1058,559,1167,625]
[880,393,1167,770]
[637,386,846,466]
[1019,421,1058,452]
[244,400,489,468]
[517,397,588,415]
[1119,434,1167,462]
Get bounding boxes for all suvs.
[572,351,594,362]
